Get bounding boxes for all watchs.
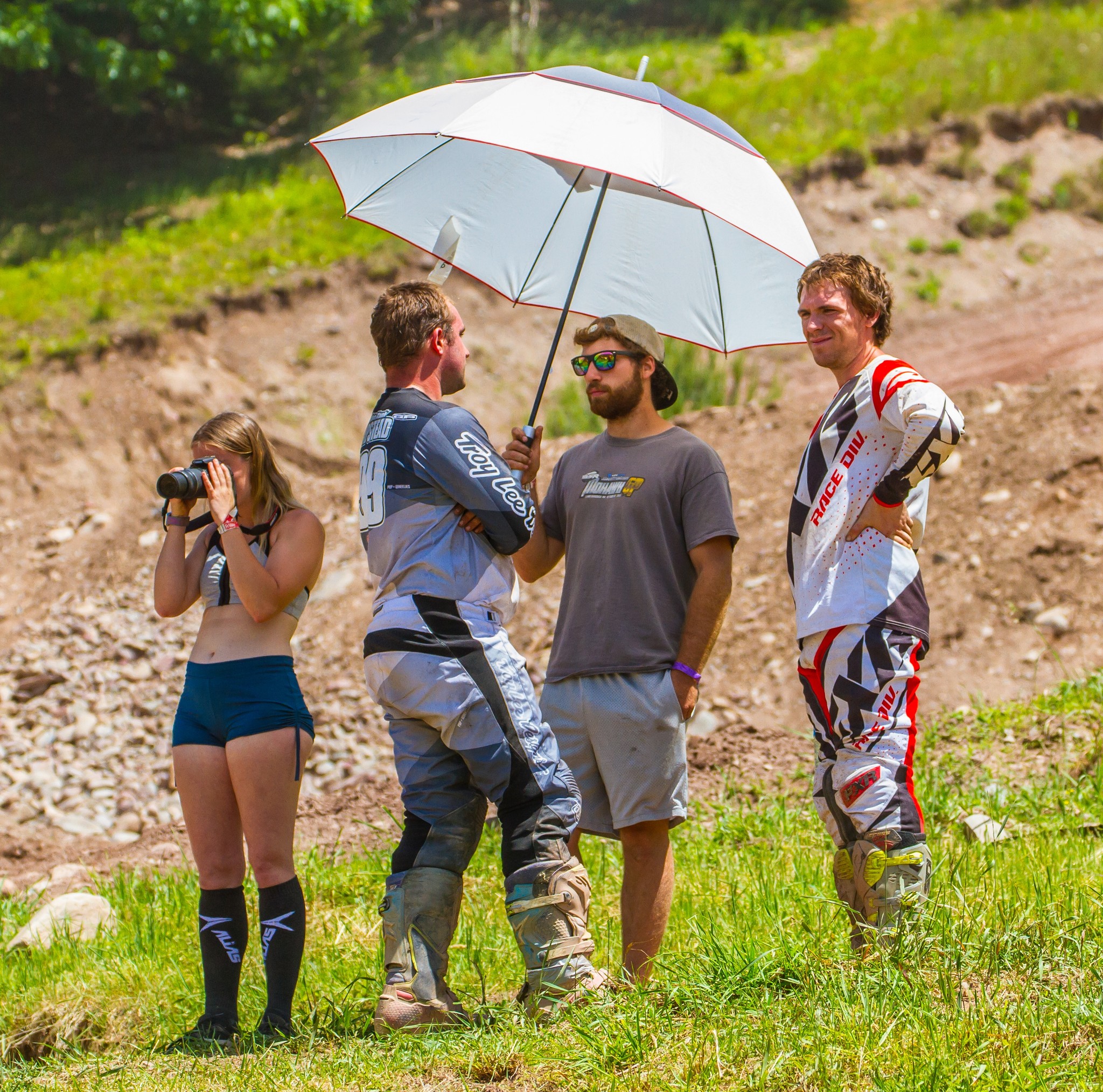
[164,511,190,527]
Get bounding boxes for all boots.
[831,829,935,956]
[505,855,613,1013]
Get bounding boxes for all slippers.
[374,866,481,1036]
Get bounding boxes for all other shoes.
[181,1012,242,1046]
[253,1009,293,1044]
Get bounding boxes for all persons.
[150,411,326,1055]
[498,312,742,1012]
[785,249,964,982]
[356,281,665,1034]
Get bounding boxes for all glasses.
[570,349,632,377]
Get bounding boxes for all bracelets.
[872,492,905,508]
[520,475,539,490]
[217,506,240,534]
[672,661,703,685]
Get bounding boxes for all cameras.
[156,455,236,499]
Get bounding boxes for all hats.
[587,314,678,411]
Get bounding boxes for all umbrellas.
[302,56,823,481]
